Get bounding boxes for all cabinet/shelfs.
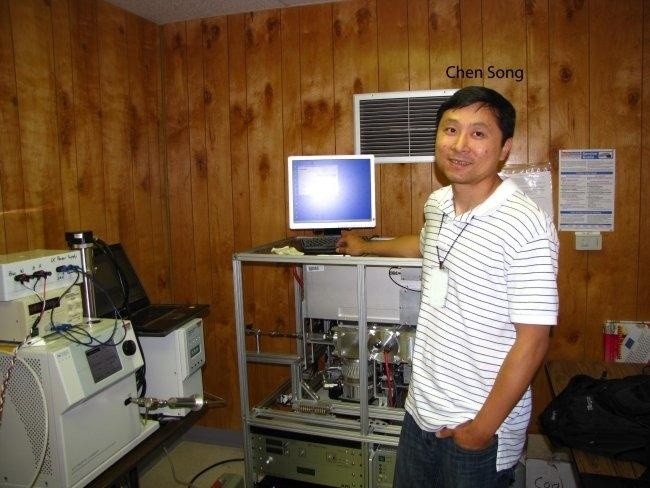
[232,237,434,486]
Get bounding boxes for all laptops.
[93,243,209,337]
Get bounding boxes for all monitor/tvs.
[287,154,377,236]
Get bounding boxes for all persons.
[332,82,561,487]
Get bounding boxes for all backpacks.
[536,367,650,466]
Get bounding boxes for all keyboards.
[301,236,342,253]
